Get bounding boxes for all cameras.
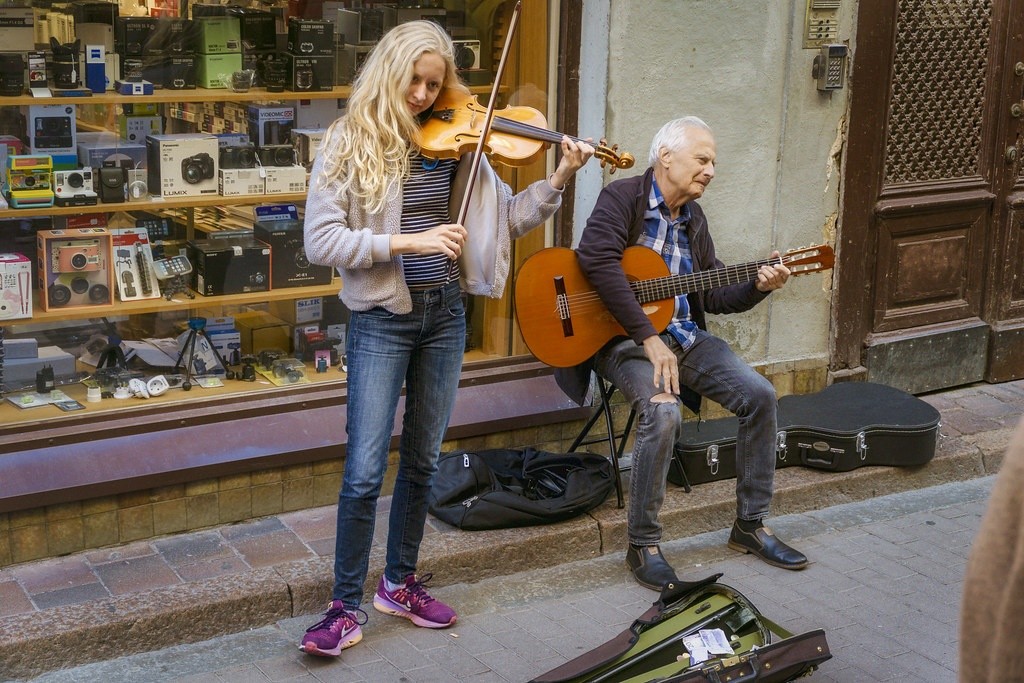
[127,169,148,201]
[53,170,93,197]
[58,244,101,273]
[6,154,55,208]
[34,116,71,136]
[181,153,214,184]
[257,145,294,167]
[219,146,255,169]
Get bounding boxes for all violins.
[410,88,635,175]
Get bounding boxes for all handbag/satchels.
[428,445,619,532]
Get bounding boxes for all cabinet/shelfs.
[1,86,512,433]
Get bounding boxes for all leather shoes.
[625,544,681,591]
[727,520,809,570]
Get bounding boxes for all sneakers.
[373,573,458,629]
[297,600,368,657]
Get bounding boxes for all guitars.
[513,242,837,368]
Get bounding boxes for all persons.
[298,18,596,658]
[554,116,809,593]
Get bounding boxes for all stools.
[567,363,694,510]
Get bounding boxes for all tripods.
[165,275,196,301]
[170,316,235,391]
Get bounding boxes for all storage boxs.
[7,8,386,294]
[2,338,76,382]
[205,297,323,366]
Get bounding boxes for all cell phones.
[150,254,193,281]
